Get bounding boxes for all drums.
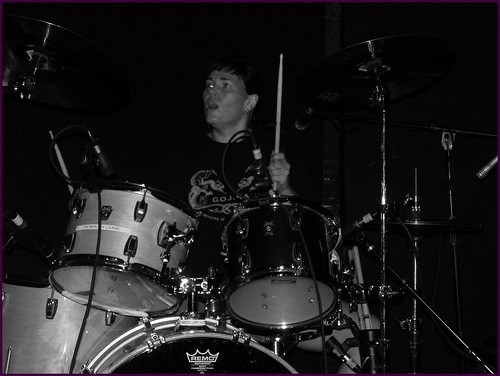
[47,176,199,317]
[172,275,223,317]
[214,195,343,329]
[1,278,142,375]
[81,316,302,375]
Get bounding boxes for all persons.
[101,52,303,280]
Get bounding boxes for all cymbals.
[364,218,483,239]
[296,34,457,118]
[1,13,133,115]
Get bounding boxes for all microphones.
[87,131,115,177]
[326,336,364,374]
[249,134,271,180]
[342,208,381,236]
[11,213,58,262]
[294,104,315,131]
[476,155,499,179]
